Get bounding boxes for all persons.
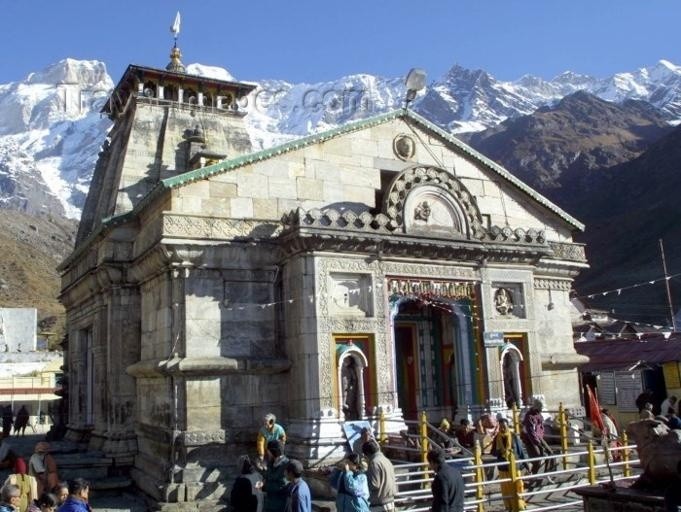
[490,418,531,512]
[283,459,311,512]
[426,450,464,512]
[353,426,375,457]
[635,388,680,429]
[325,454,370,512]
[256,414,286,463]
[16,404,29,439]
[253,441,289,512]
[521,399,554,490]
[438,413,499,481]
[600,409,619,460]
[228,455,264,512]
[2,404,13,437]
[361,439,396,512]
[0,436,91,512]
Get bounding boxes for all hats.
[440,418,451,429]
[283,459,305,476]
[533,399,544,409]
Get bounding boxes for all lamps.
[405,69,426,110]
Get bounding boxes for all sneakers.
[544,475,555,485]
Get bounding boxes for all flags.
[586,384,604,433]
